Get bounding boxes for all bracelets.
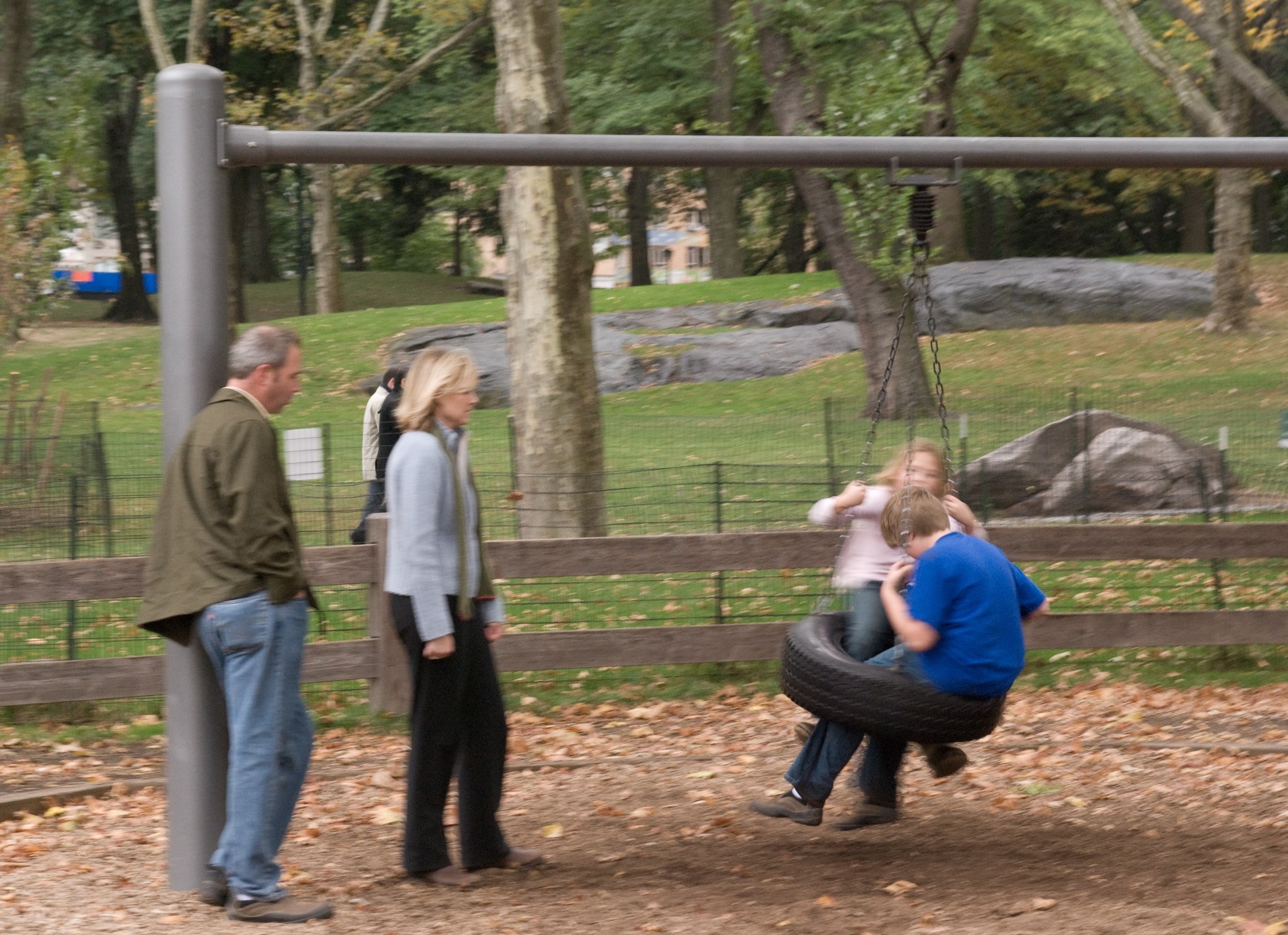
[963,520,982,534]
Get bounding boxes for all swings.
[779,239,1009,746]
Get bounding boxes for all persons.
[745,484,1049,830]
[347,366,409,545]
[137,324,335,925]
[379,345,542,891]
[792,432,991,778]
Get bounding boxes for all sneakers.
[226,893,333,923]
[751,793,822,825]
[198,866,229,907]
[836,802,897,828]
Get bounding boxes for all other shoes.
[466,846,544,871]
[925,746,966,778]
[794,722,814,743]
[409,860,482,884]
[349,532,366,544]
